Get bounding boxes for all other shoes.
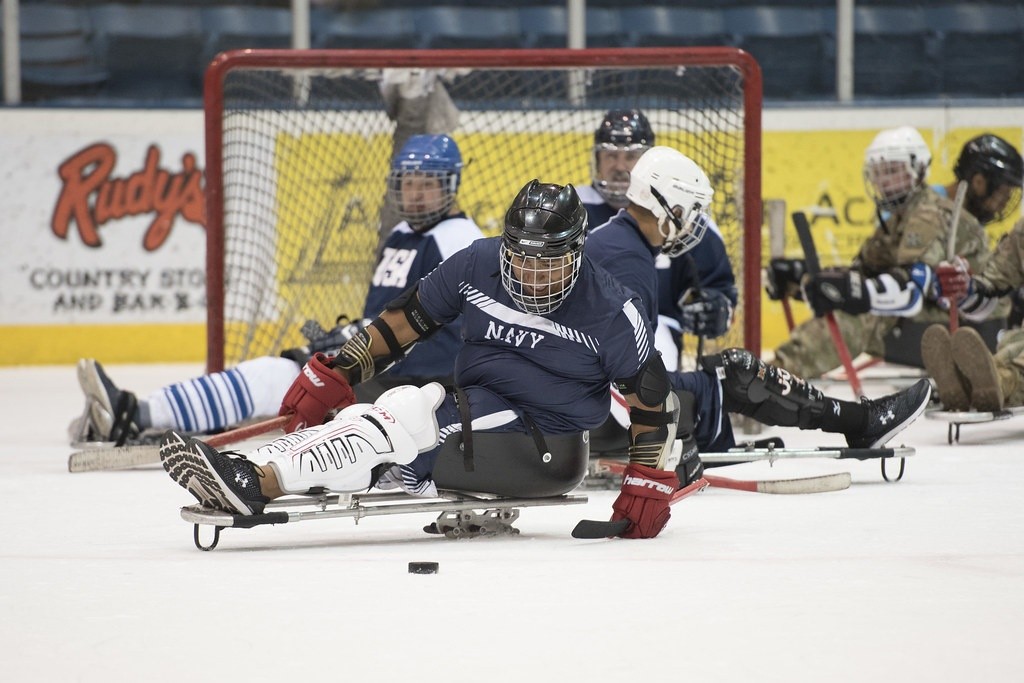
[920,324,971,414]
[951,327,1004,414]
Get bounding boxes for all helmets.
[954,134,1024,185]
[394,134,461,198]
[866,126,932,174]
[503,179,588,258]
[594,107,656,149]
[626,145,714,221]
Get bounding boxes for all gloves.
[937,257,972,297]
[800,272,869,320]
[676,287,731,338]
[608,463,678,540]
[762,258,814,302]
[279,352,354,435]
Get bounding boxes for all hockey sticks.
[571,479,705,539]
[701,469,850,494]
[792,209,866,400]
[67,414,291,473]
[768,198,794,331]
[949,179,967,331]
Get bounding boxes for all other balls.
[410,562,438,573]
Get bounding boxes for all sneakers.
[159,432,270,520]
[70,359,143,447]
[847,379,933,460]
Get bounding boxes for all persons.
[763,125,1024,408]
[77,134,483,441]
[581,144,932,453]
[159,179,679,540]
[575,107,739,368]
